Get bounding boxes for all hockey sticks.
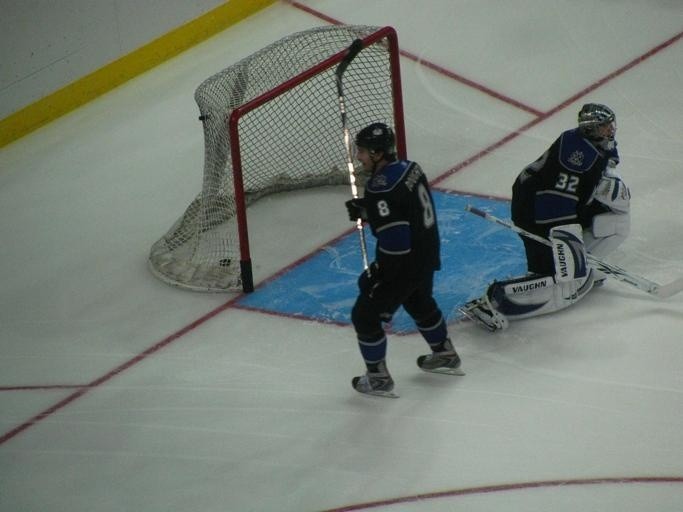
[336,39,373,280]
[464,203,683,298]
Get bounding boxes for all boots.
[466,293,509,330]
[351,372,394,393]
[418,336,462,369]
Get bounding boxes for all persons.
[457,102,632,336]
[344,123,465,398]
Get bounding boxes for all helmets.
[354,123,394,151]
[578,103,616,140]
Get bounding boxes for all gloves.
[358,268,391,297]
[345,198,366,221]
[594,177,630,211]
